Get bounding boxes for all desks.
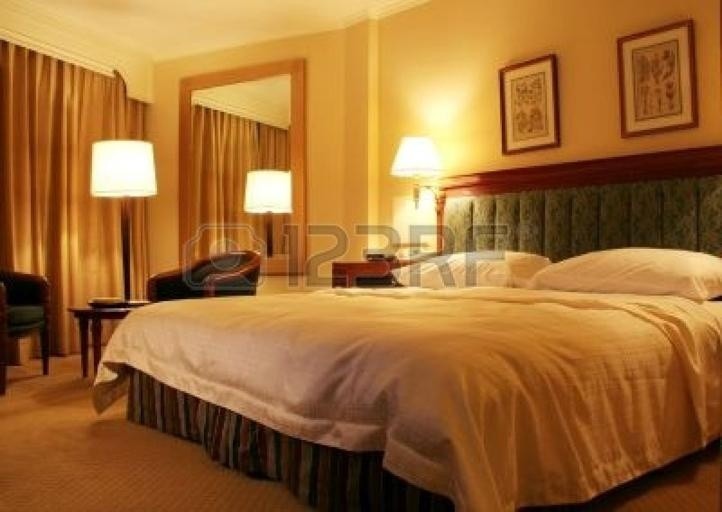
[68,306,141,381]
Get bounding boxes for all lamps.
[243,169,295,276]
[88,139,158,310]
[387,135,441,260]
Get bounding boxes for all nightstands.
[331,262,403,288]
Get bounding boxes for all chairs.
[146,251,262,305]
[0,267,53,394]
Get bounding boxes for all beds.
[128,147,722,509]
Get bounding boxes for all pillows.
[393,250,551,286]
[528,246,722,302]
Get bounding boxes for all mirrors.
[171,55,314,279]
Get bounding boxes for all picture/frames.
[616,19,700,141]
[497,54,560,156]
